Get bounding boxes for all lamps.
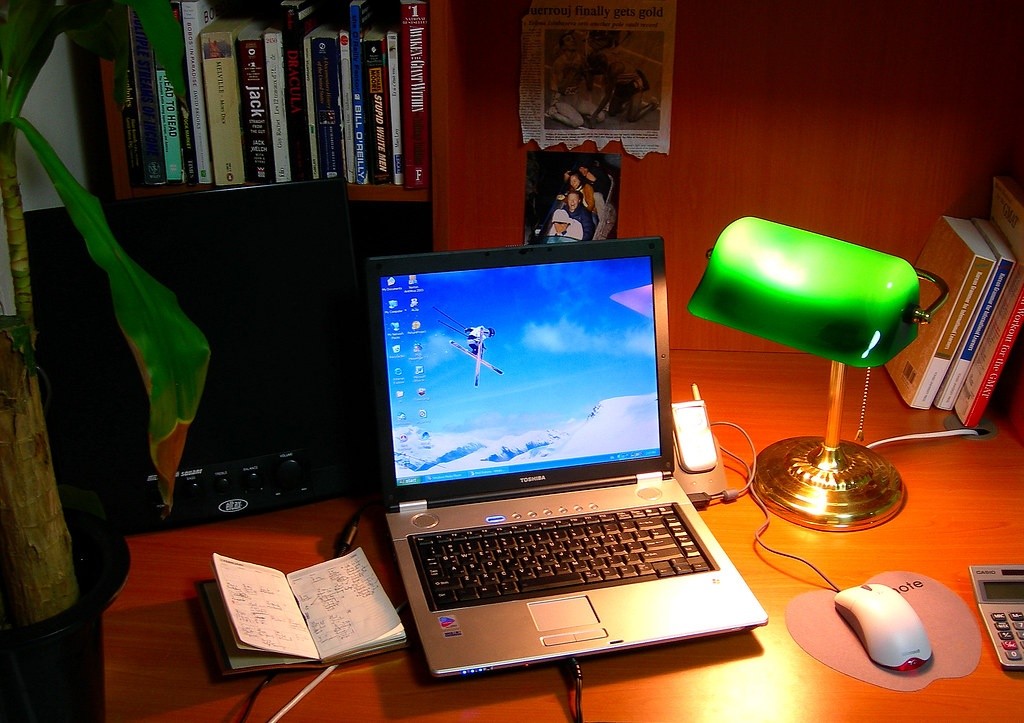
[687,215,949,533]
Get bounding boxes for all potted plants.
[0,0,213,723]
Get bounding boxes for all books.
[883,176,1024,427]
[123,0,432,190]
[197,546,411,676]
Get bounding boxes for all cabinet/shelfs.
[100,0,465,254]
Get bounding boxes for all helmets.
[489,327,495,337]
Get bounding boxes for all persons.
[544,29,659,129]
[464,325,494,359]
[543,153,609,243]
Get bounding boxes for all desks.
[103,346,1024,723]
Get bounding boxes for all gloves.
[464,328,469,333]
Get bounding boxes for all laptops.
[367,234,768,678]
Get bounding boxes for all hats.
[552,209,569,223]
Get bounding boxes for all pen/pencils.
[266,603,404,723]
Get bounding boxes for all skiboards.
[448,325,505,389]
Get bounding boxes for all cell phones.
[671,383,717,473]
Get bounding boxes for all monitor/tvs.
[21,177,366,536]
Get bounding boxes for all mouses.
[835,583,932,672]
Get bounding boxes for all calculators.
[968,564,1024,672]
